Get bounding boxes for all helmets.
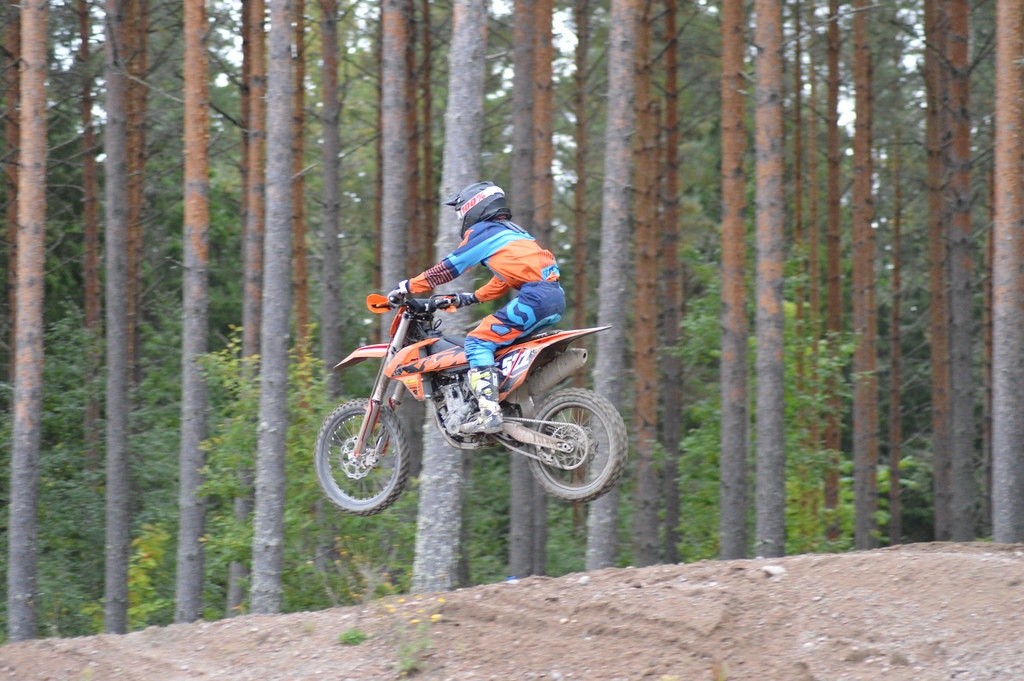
[446,181,511,240]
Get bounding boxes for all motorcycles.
[315,285,631,517]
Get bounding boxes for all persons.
[387,180,567,434]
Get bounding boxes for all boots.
[459,365,503,434]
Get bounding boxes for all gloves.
[387,279,410,307]
[454,292,480,308]
